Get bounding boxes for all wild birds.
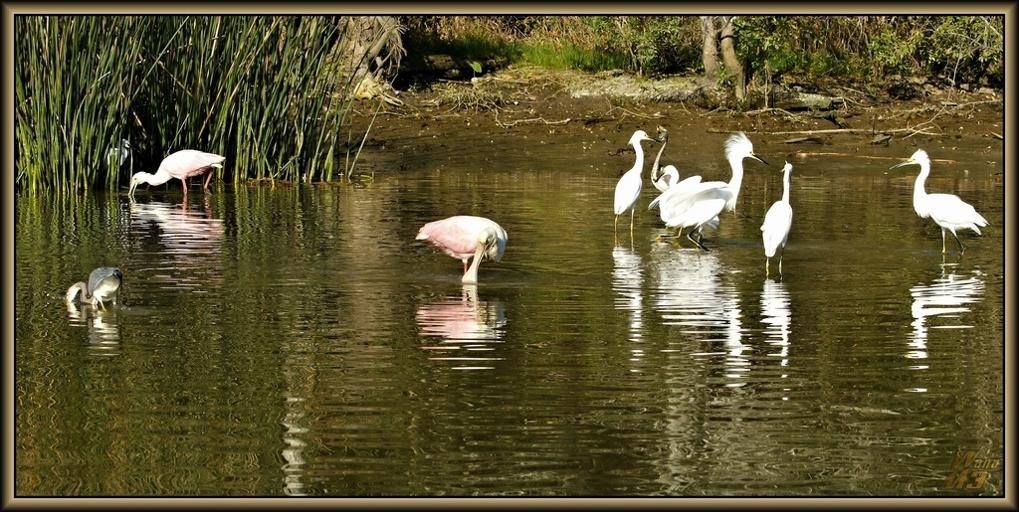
[65,266,123,315]
[613,122,794,275]
[415,215,509,299]
[126,150,225,209]
[889,148,991,255]
